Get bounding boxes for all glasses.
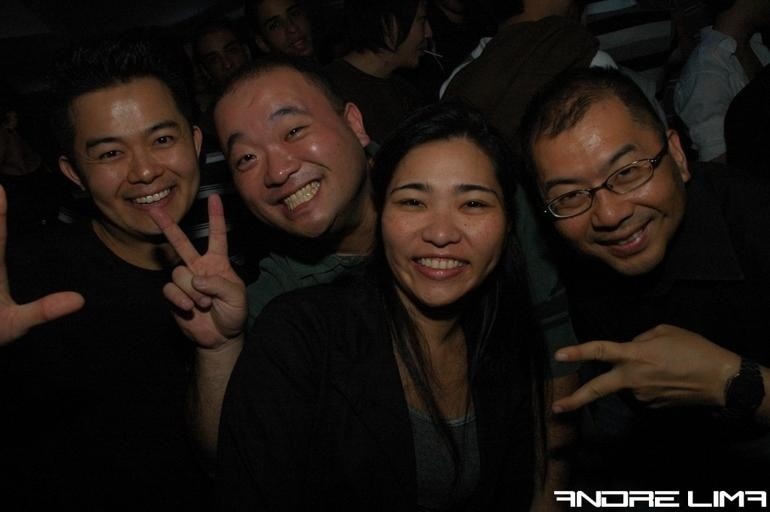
[543,137,669,222]
